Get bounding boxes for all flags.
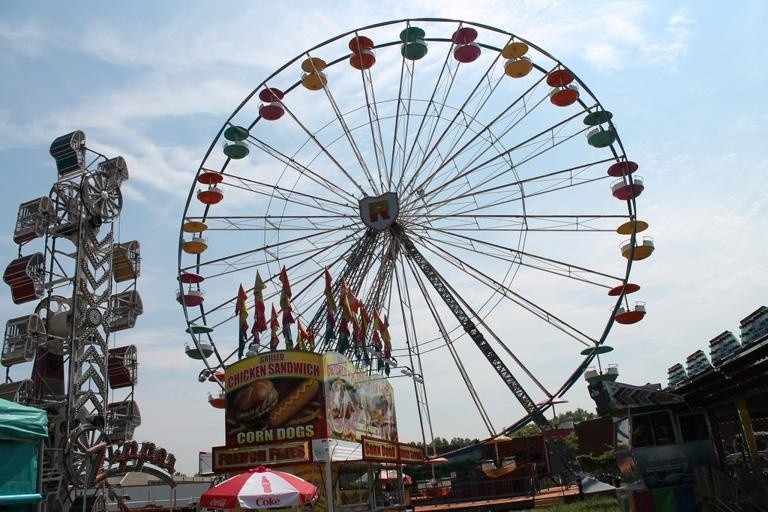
[235,265,392,378]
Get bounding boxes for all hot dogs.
[267,379,320,429]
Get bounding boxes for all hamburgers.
[232,379,280,421]
